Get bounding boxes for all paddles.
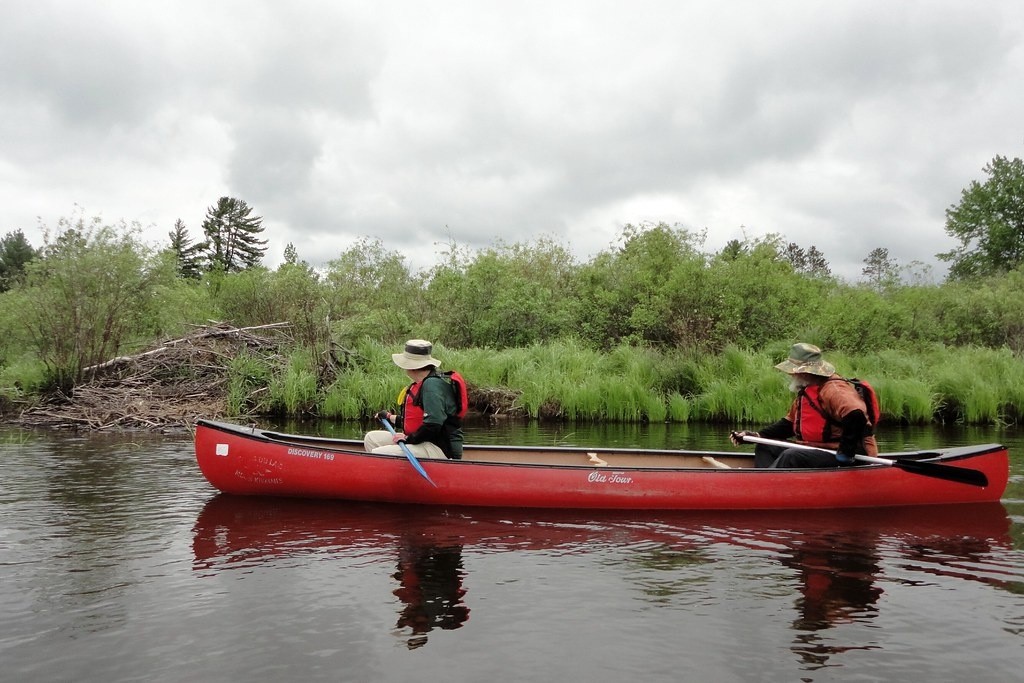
[732,429,989,488]
[376,416,439,489]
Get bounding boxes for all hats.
[774,342,836,377]
[391,339,441,369]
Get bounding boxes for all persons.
[363,339,464,460]
[728,342,879,470]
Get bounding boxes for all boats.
[191,492,1016,568]
[193,417,1010,507]
